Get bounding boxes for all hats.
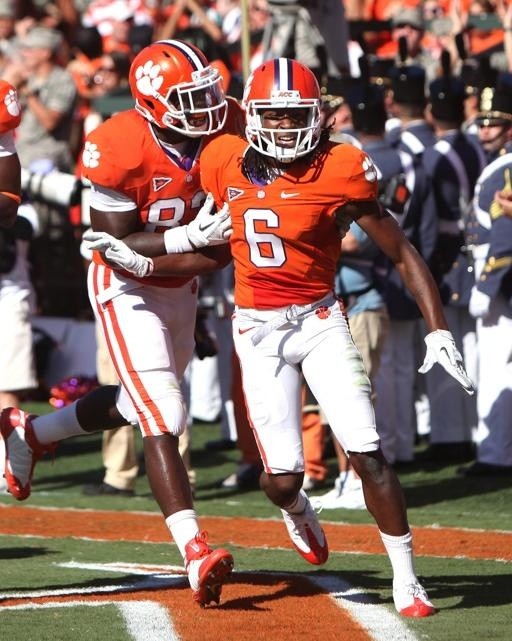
[392,10,423,29]
[346,54,511,129]
[22,27,60,47]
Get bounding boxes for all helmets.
[129,40,229,139]
[240,57,323,165]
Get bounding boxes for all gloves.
[417,328,476,396]
[164,193,234,254]
[81,232,151,279]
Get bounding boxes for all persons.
[0,39,247,609]
[81,57,476,617]
[1,0,512,509]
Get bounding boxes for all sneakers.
[0,406,59,501]
[279,489,328,565]
[310,475,366,510]
[83,484,134,496]
[392,578,434,618]
[181,530,234,609]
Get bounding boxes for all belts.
[235,294,336,346]
